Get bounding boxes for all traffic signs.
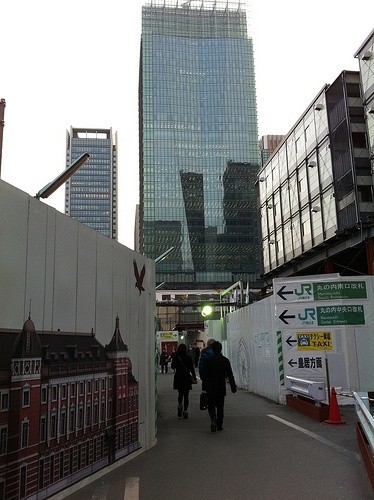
[287,354,324,371]
[273,280,369,304]
[284,331,335,351]
[275,303,367,330]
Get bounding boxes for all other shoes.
[177,406,188,419]
[202,407,224,433]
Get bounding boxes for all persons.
[199,338,237,432]
[187,344,200,367]
[171,343,197,419]
[155,348,169,374]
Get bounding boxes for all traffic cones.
[322,386,347,425]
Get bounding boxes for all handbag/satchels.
[190,374,197,384]
[199,392,208,410]
